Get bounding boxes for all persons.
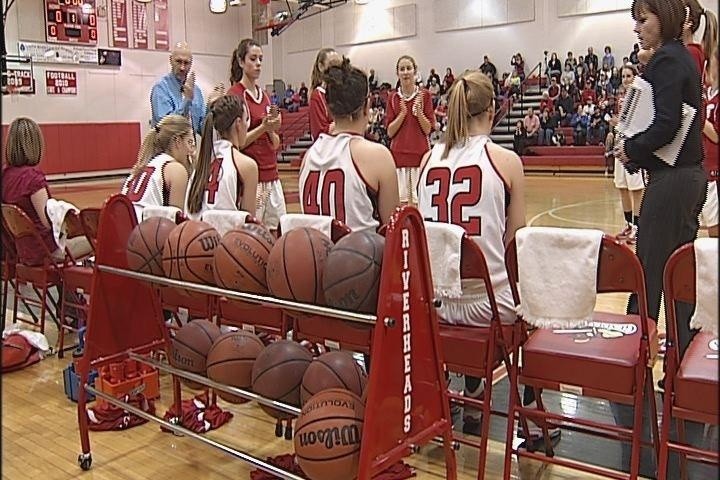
[309,47,342,140]
[270,81,310,114]
[183,94,258,218]
[120,113,196,209]
[297,67,400,243]
[610,0,700,391]
[697,45,718,235]
[367,56,455,207]
[479,43,640,153]
[678,0,718,133]
[223,39,287,242]
[416,72,563,442]
[612,63,637,242]
[1,119,87,322]
[150,41,206,138]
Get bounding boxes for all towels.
[513,227,604,331]
[691,239,718,340]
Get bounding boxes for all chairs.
[659,242,718,480]
[503,236,660,480]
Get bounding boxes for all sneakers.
[447,379,493,423]
[57,293,87,318]
[616,220,638,245]
[517,401,561,439]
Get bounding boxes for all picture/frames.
[98,48,121,65]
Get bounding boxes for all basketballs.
[322,229,386,331]
[294,389,363,480]
[265,226,337,319]
[171,319,223,390]
[161,217,224,298]
[299,348,370,405]
[251,338,316,420]
[207,330,267,405]
[126,217,177,288]
[212,221,278,309]
